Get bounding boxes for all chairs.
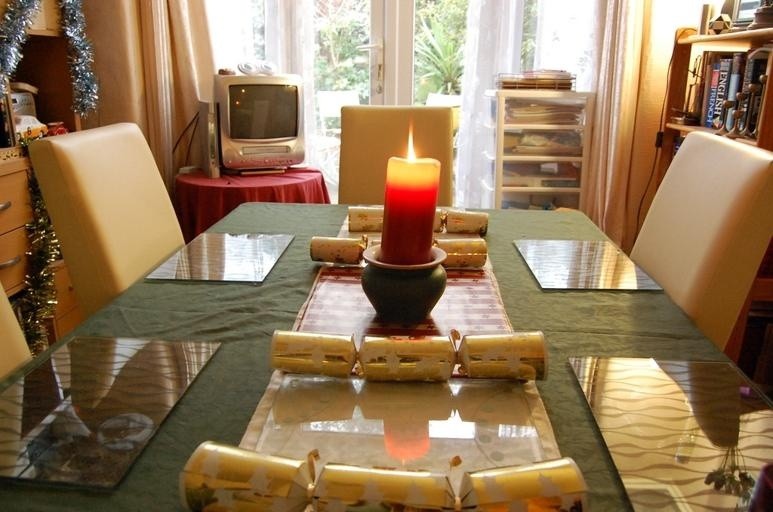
[0,279,33,386]
[28,122,186,317]
[628,130,773,353]
[338,104,454,207]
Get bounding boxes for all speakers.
[196,98,221,179]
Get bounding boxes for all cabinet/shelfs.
[0,169,35,292]
[43,259,82,346]
[658,26,773,322]
[480,89,597,213]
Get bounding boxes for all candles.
[383,419,430,461]
[379,156,441,265]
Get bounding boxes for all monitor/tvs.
[212,74,305,175]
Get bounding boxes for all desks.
[0,201,769,512]
[174,167,331,245]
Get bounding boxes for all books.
[698,50,768,137]
[502,77,583,188]
